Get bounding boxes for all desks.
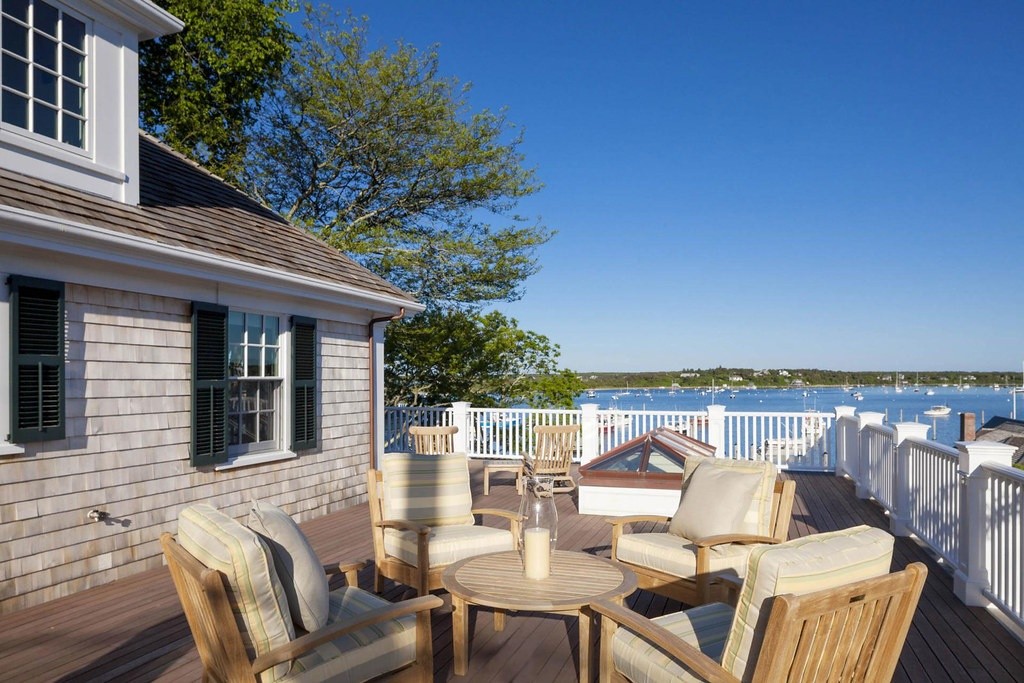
[444,549,639,683]
[482,460,524,496]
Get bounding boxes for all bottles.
[516,474,558,569]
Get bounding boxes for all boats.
[666,405,691,435]
[597,401,634,434]
[922,404,952,415]
[758,422,811,458]
[800,395,827,437]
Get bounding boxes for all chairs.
[605,455,796,605]
[523,424,580,493]
[158,503,443,683]
[366,453,525,597]
[589,523,928,683]
[408,426,459,454]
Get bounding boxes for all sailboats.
[584,370,1024,402]
[688,377,716,427]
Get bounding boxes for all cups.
[524,528,549,580]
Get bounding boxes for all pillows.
[248,498,330,634]
[668,460,763,556]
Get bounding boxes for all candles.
[524,528,550,579]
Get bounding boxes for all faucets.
[87,509,100,523]
